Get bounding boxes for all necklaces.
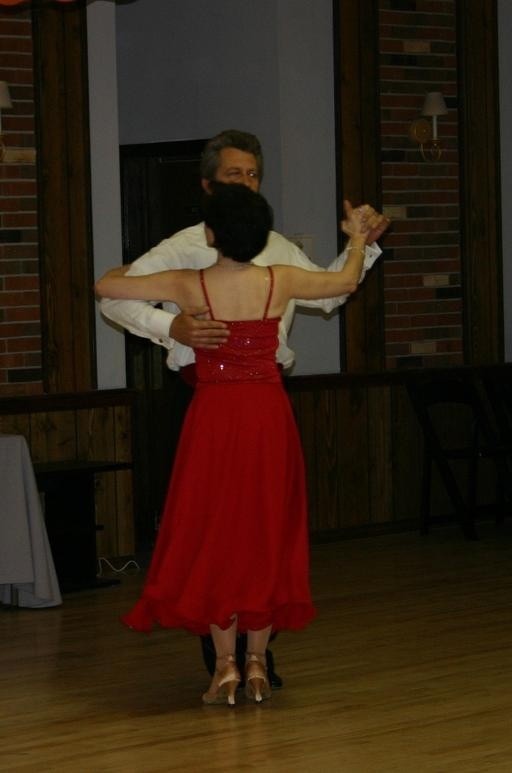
[210,255,254,273]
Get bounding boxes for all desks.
[403,362,511,541]
[0,434,64,609]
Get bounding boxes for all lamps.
[0,82,14,131]
[419,92,449,164]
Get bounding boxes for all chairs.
[34,459,133,588]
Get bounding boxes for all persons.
[100,128,391,691]
[346,246,367,255]
[95,182,377,707]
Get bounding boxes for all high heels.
[202,653,241,708]
[243,653,272,704]
